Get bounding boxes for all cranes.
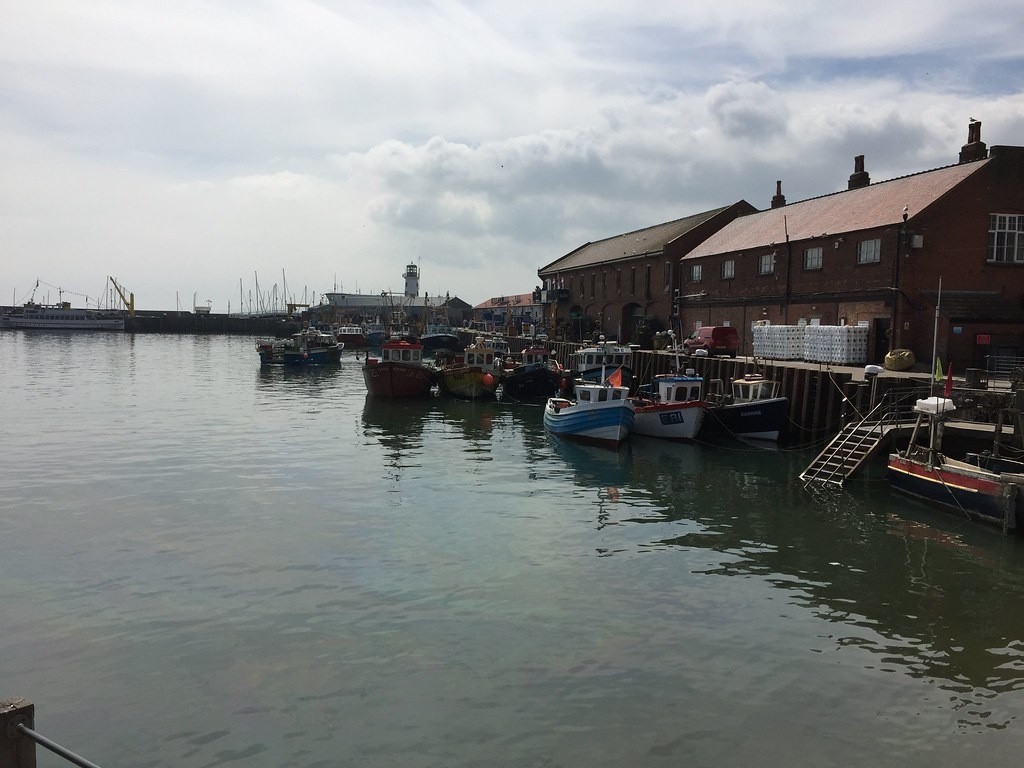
[109,277,134,316]
[175,269,393,321]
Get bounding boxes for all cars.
[684,326,741,357]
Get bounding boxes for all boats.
[1,301,125,330]
[338,292,461,348]
[258,320,344,366]
[886,361,1024,531]
[361,332,561,404]
[542,344,788,450]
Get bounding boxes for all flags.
[936,356,943,382]
[608,368,621,387]
[944,363,953,397]
